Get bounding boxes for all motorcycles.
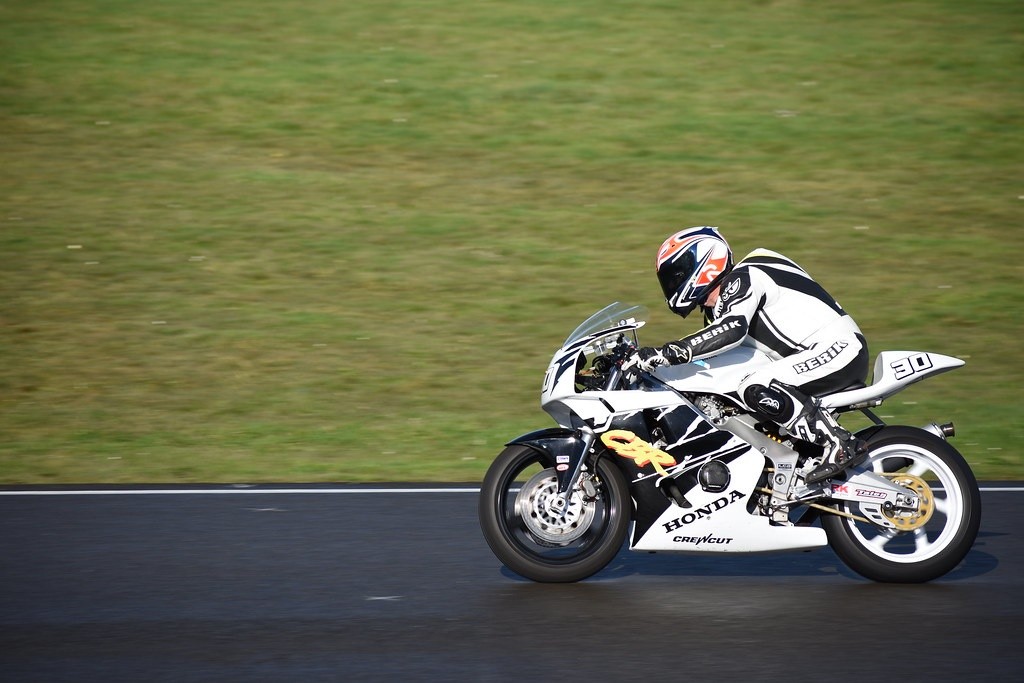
[476,299,983,584]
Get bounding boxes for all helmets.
[656,225,735,319]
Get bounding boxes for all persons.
[618,225,869,484]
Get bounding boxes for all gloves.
[621,340,693,373]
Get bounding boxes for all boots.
[785,395,869,482]
